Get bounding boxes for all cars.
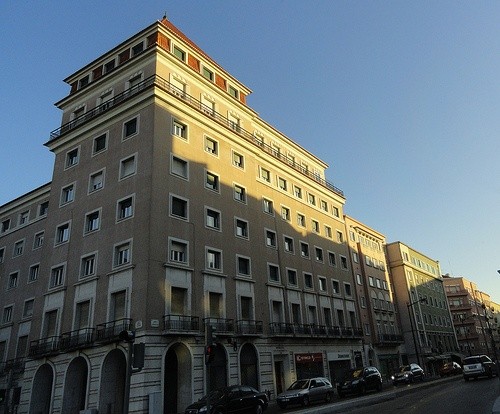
[276,377,334,409]
[184,382,270,414]
[439,361,462,377]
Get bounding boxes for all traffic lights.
[120,329,135,345]
[206,345,213,356]
[207,324,218,349]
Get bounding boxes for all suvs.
[461,354,500,382]
[390,364,425,386]
[334,367,384,399]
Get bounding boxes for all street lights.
[407,298,427,368]
